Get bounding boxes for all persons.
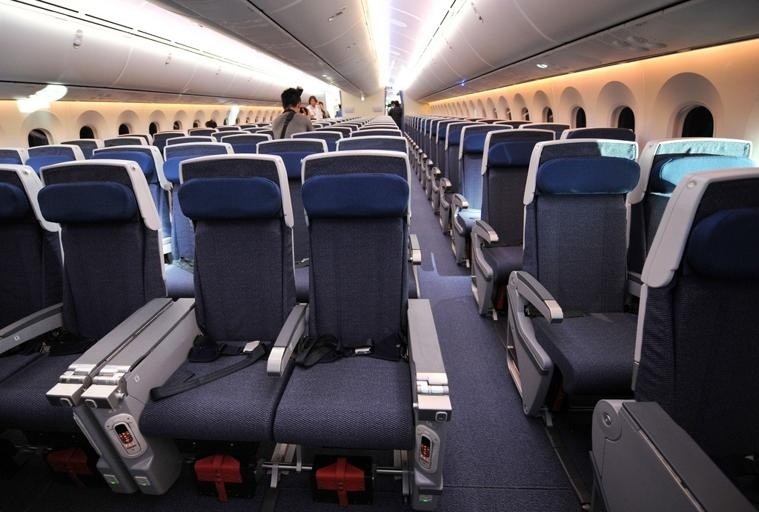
[306,95,323,120]
[271,88,313,140]
[319,101,331,118]
[387,100,402,130]
[335,104,345,117]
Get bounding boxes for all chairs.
[625,137,753,273]
[162,140,236,299]
[90,144,173,237]
[0,162,66,381]
[80,153,300,507]
[589,167,759,512]
[336,136,422,299]
[256,137,330,299]
[264,149,454,511]
[506,138,642,417]
[0,157,176,496]
[26,144,86,180]
[0,147,28,165]
